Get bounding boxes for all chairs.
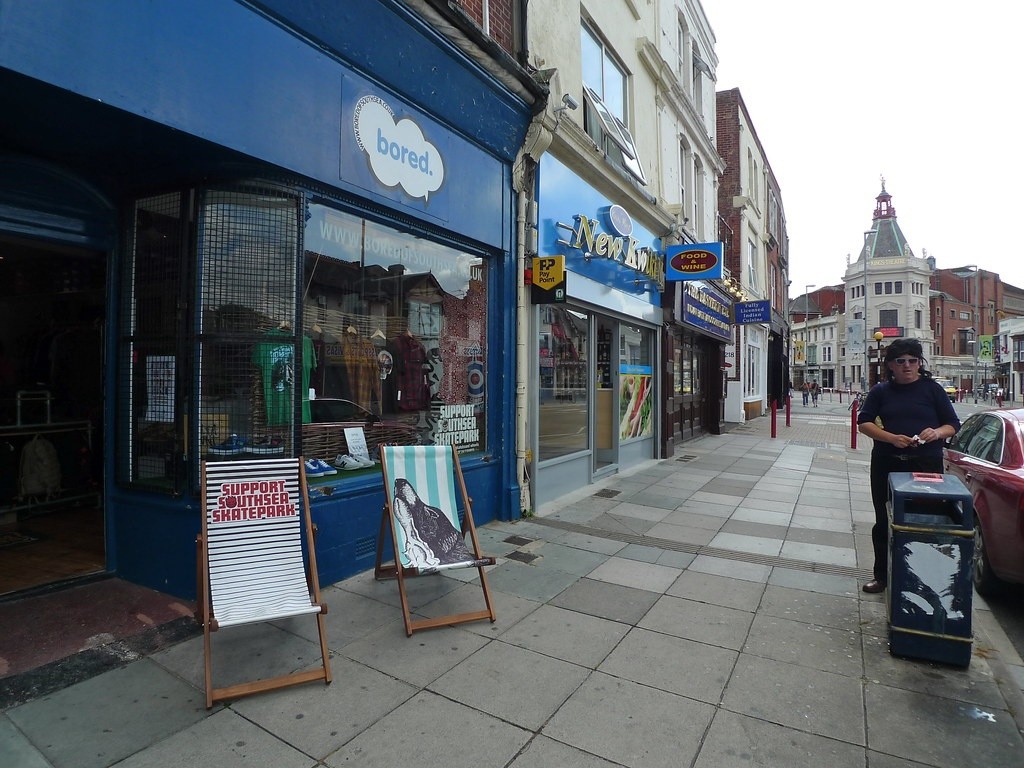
[375,443,500,637]
[199,457,332,709]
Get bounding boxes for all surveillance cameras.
[562,94,579,111]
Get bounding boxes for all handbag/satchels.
[811,388,816,395]
[17,434,62,508]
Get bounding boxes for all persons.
[856,340,960,593]
[955,382,1010,405]
[786,380,819,408]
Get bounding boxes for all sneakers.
[370,443,387,464]
[242,435,286,455]
[388,442,397,446]
[208,433,240,455]
[352,455,375,468]
[304,458,325,477]
[334,453,364,470]
[314,458,337,475]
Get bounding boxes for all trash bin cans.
[884,471,977,669]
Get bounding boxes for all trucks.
[931,376,956,404]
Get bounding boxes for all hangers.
[402,324,414,337]
[347,324,358,334]
[313,322,322,334]
[277,318,295,332]
[371,322,388,342]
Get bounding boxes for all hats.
[885,338,922,361]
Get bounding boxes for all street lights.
[806,284,816,383]
[967,340,977,404]
[873,332,884,384]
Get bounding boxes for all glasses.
[893,357,919,366]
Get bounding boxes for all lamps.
[732,282,744,298]
[730,276,737,295]
[723,274,732,287]
[740,288,749,303]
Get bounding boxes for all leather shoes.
[862,578,885,593]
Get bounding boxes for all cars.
[939,408,1024,600]
[976,382,1007,402]
